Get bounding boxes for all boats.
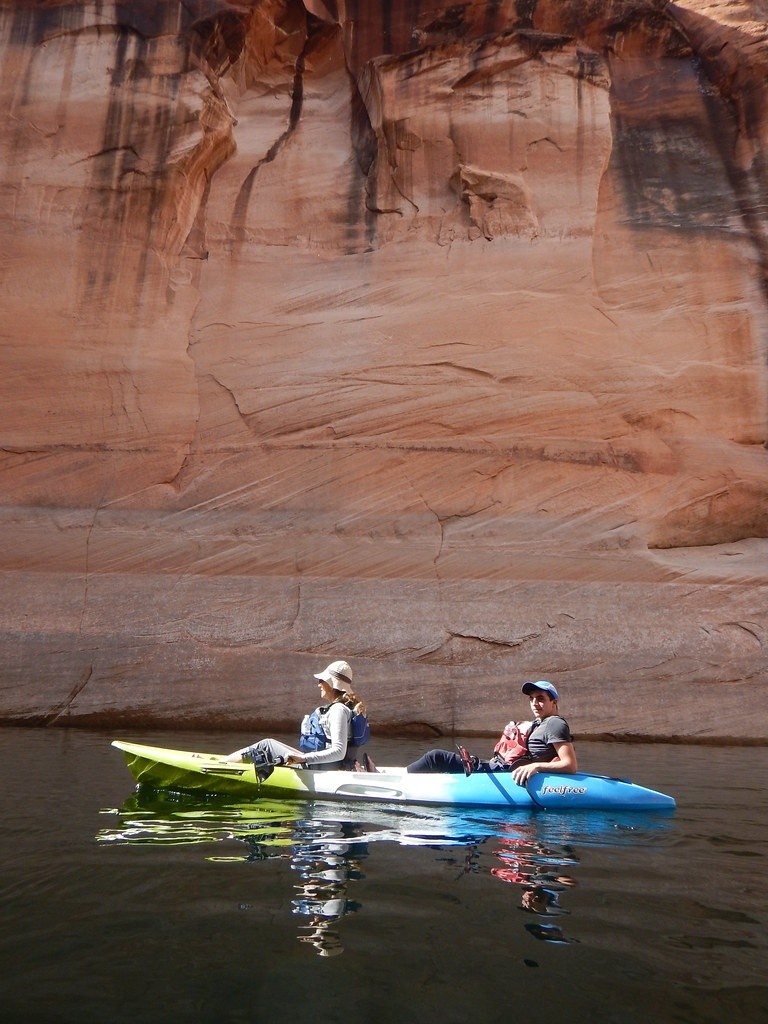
[110,738,678,812]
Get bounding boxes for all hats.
[520,680,559,701]
[314,661,354,695]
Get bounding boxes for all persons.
[353,680,578,787]
[191,661,368,771]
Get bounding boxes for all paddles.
[456,743,481,778]
[253,746,289,784]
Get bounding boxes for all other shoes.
[353,759,365,773]
[363,753,379,773]
